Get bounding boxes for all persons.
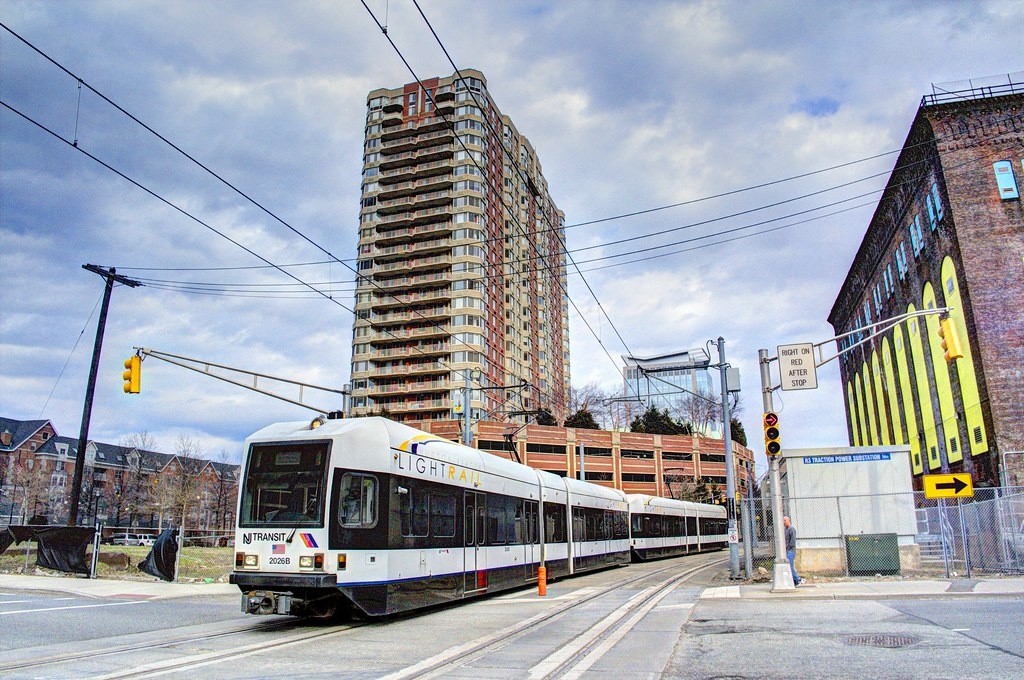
[783,517,803,587]
[343,482,366,523]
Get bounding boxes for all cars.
[136,533,158,547]
[100,535,114,545]
[110,532,139,546]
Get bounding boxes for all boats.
[721,493,726,503]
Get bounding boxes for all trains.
[228,410,730,618]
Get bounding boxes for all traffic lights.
[762,412,782,456]
[123,355,141,393]
[715,500,719,504]
[938,318,963,363]
[735,492,741,501]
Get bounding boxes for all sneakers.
[795,578,803,588]
